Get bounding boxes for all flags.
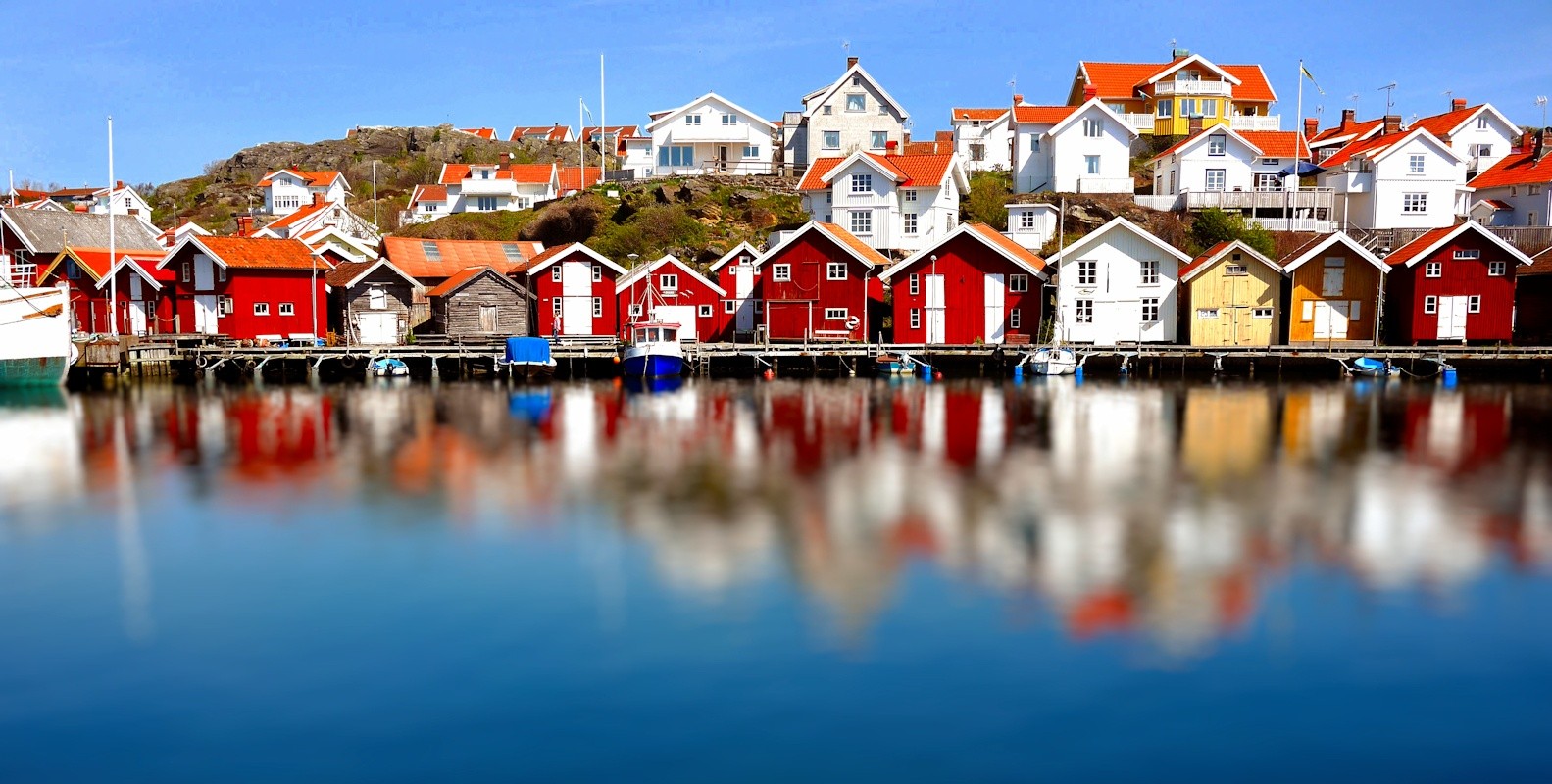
[1302,67,1325,95]
[583,101,597,129]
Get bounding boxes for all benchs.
[255,334,288,346]
[288,332,320,347]
[812,330,850,344]
[1005,334,1031,351]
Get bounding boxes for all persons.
[550,312,567,347]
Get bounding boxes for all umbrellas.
[1275,158,1328,191]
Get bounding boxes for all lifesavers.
[846,315,860,330]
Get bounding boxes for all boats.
[497,352,559,382]
[0,273,73,386]
[876,353,915,373]
[1026,308,1077,376]
[368,356,409,377]
[1352,355,1402,378]
[620,319,682,376]
